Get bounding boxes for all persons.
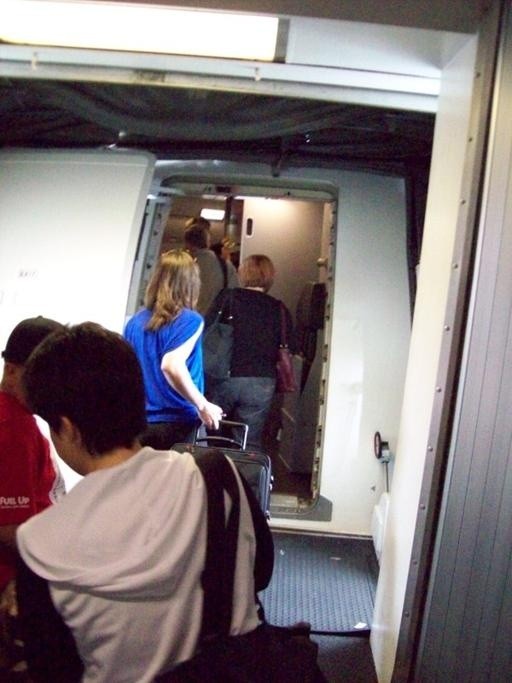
[0,316,66,683]
[202,254,297,450]
[184,224,239,315]
[16,321,274,683]
[184,216,224,257]
[124,249,223,450]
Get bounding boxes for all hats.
[2,315,68,363]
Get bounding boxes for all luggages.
[171,415,274,522]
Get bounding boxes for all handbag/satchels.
[150,449,328,683]
[202,287,235,381]
[277,300,298,393]
